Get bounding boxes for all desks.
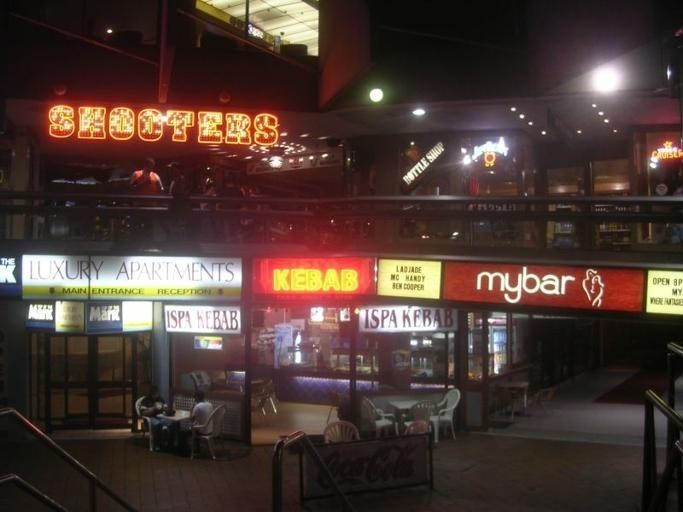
[155,409,191,454]
[494,379,529,416]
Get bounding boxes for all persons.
[138,384,170,453]
[175,391,214,457]
[167,162,272,243]
[130,157,166,207]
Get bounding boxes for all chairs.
[190,405,227,461]
[532,387,559,417]
[135,395,155,454]
[488,388,517,420]
[323,388,461,447]
[191,368,277,415]
